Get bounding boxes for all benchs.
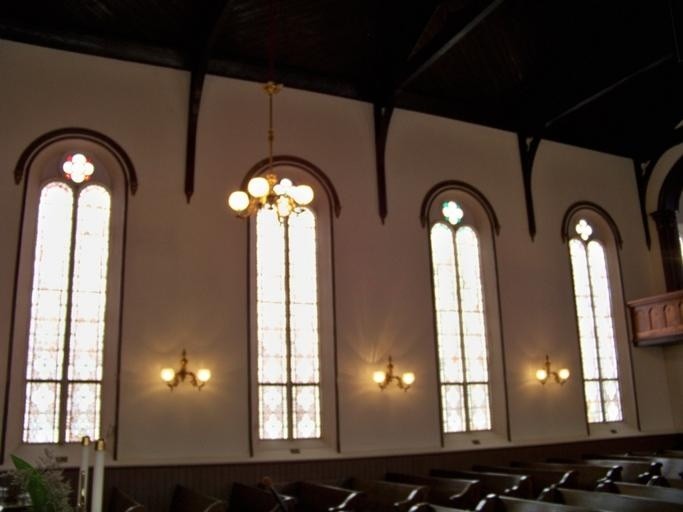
[108,447,683,512]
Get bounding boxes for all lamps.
[225,82,313,225]
[368,354,416,393]
[533,355,573,387]
[157,344,210,392]
